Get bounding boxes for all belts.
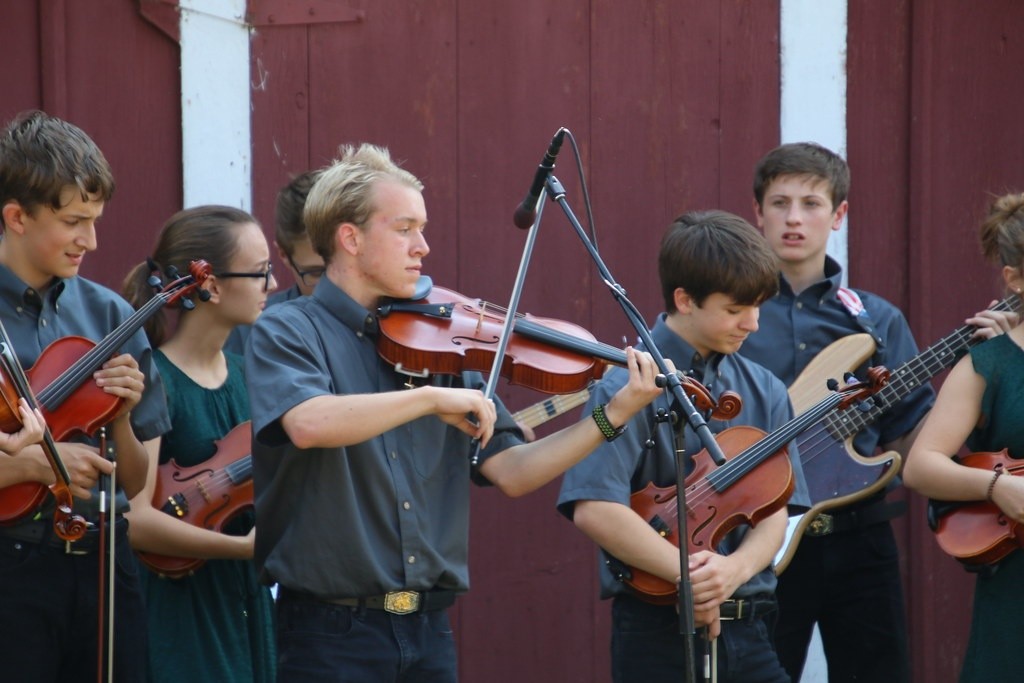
[11,518,128,555]
[801,500,887,540]
[313,589,456,616]
[718,598,776,621]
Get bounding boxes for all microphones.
[513,128,564,230]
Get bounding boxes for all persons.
[737,143,1019,682]
[100,204,282,683]
[0,109,173,683]
[226,166,328,361]
[243,141,676,683]
[901,192,1023,683]
[555,207,812,683]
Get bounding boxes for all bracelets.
[988,471,1004,501]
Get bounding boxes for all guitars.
[768,291,1024,578]
[510,329,655,429]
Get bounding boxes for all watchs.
[591,403,627,441]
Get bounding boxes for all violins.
[378,276,742,423]
[0,257,212,525]
[0,320,87,541]
[600,362,891,604]
[130,419,255,579]
[927,447,1024,573]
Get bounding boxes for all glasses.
[215,265,272,291]
[287,255,323,287]
